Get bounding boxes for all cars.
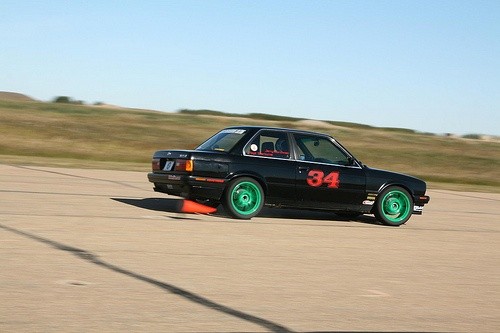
[146,125,430,224]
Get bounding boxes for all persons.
[276,137,290,158]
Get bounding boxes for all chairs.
[261,137,289,158]
